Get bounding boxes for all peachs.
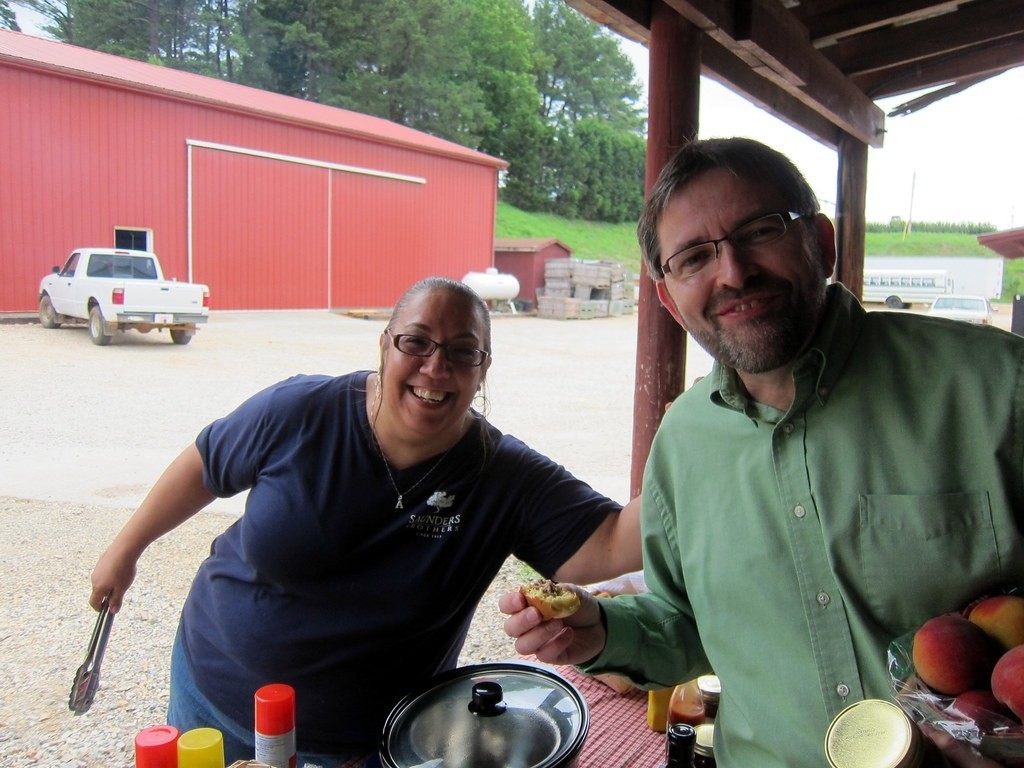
[912,594,1024,734]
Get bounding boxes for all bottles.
[665,675,704,739]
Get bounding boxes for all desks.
[340,652,714,768]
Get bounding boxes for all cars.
[929,294,994,326]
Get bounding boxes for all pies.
[519,578,580,621]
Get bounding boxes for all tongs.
[67,593,114,716]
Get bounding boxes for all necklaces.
[371,383,466,509]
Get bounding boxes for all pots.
[380,659,591,768]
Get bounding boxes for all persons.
[500,137,1024,768]
[89,276,705,768]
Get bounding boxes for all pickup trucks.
[39,247,210,346]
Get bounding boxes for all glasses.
[661,211,805,277]
[387,330,489,367]
